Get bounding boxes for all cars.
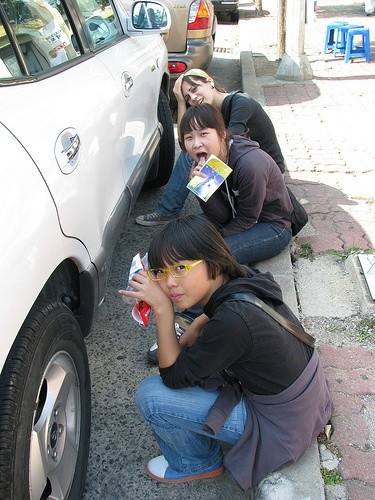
[211,0,239,24]
[0,0,173,499]
[119,0,217,110]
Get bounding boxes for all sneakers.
[147,323,186,364]
[146,455,224,482]
[136,212,175,226]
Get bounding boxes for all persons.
[117,214,332,492]
[136,69,284,229]
[146,103,294,366]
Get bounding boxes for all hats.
[184,69,209,79]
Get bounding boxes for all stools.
[324,21,371,63]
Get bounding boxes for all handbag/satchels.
[286,186,308,236]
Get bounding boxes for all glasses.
[147,259,203,281]
